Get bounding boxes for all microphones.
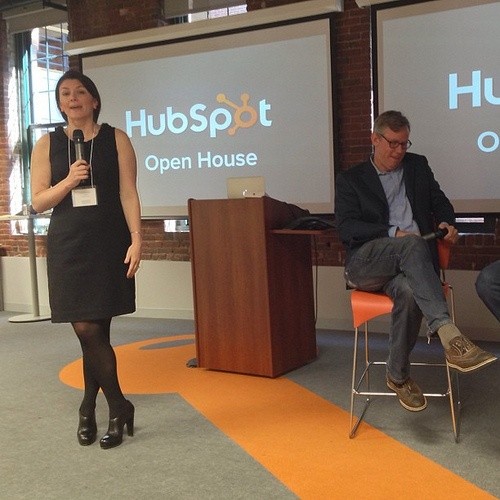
[422,228,449,240]
[73,129,88,184]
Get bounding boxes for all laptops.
[226,176,265,198]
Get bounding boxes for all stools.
[349,236,462,443]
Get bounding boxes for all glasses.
[379,132,412,149]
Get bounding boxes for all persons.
[335,110,498,413]
[475,259,500,321]
[30,69,143,450]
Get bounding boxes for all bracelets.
[130,231,144,237]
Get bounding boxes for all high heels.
[77,401,97,446]
[100,399,135,448]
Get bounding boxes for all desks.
[0,210,51,322]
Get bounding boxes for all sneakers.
[445,333,498,372]
[386,374,427,412]
